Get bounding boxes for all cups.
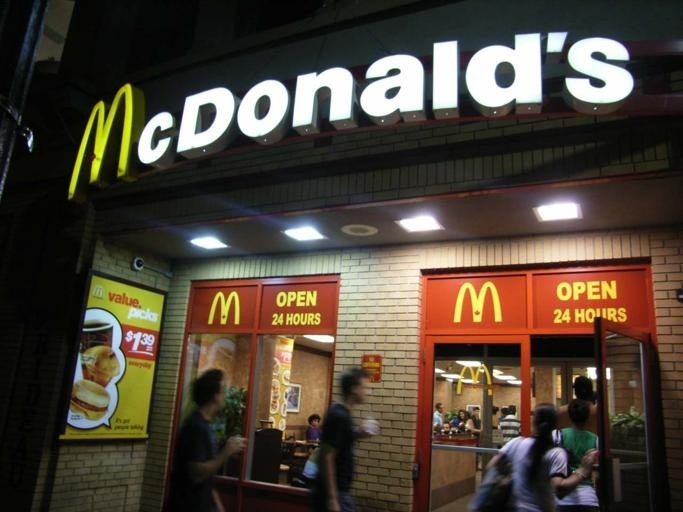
[81,324,113,352]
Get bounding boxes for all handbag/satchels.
[468,468,511,511]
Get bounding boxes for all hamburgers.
[70,379,110,420]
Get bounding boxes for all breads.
[81,346,120,387]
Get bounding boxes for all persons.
[432,376,600,512]
[300,447,320,484]
[306,414,320,450]
[309,367,380,512]
[162,369,248,512]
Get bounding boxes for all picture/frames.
[287,383,302,413]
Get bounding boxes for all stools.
[275,439,320,482]
